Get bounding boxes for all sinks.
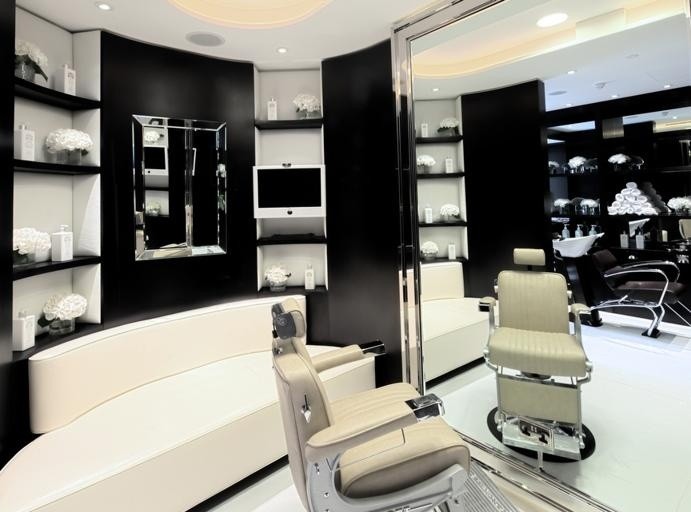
[552,231,605,257]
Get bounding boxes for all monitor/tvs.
[253,164,327,219]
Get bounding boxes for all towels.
[548,153,690,222]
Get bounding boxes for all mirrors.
[393,0,691,512]
[129,114,229,262]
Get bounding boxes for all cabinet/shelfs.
[253,117,327,294]
[0,1,102,363]
[411,139,467,260]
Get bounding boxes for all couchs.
[399,262,499,389]
[0,294,376,512]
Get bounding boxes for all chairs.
[592,248,690,338]
[270,297,471,512]
[478,248,596,473]
